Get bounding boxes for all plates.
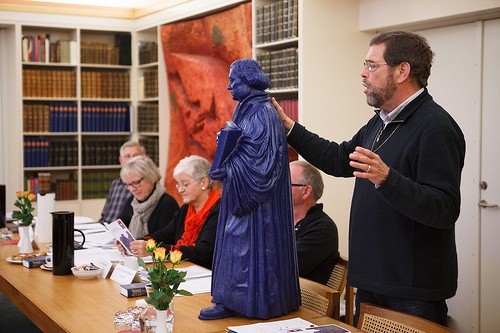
[6,253,52,264]
[40,263,53,270]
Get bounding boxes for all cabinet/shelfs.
[13,0,303,199]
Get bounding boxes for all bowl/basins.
[71,267,102,279]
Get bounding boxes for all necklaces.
[370,123,400,152]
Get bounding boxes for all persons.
[289,161,339,286]
[98,141,146,226]
[120,156,180,239]
[272,30,466,328]
[116,155,221,270]
[198,58,303,320]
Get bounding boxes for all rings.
[367,165,371,172]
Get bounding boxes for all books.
[272,100,298,162]
[120,282,147,298]
[256,0,298,44]
[23,256,51,269]
[21,35,131,201]
[137,42,159,166]
[257,47,298,90]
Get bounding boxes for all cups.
[45,254,52,267]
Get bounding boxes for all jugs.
[50,210,85,274]
[35,191,55,242]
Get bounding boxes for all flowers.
[10,191,37,225]
[137,240,193,310]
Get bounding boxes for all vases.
[19,226,34,254]
[155,310,170,333]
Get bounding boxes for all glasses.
[176,177,208,189]
[122,175,145,190]
[363,60,403,73]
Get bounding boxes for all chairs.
[299,256,457,333]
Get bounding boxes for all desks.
[0,233,366,333]
